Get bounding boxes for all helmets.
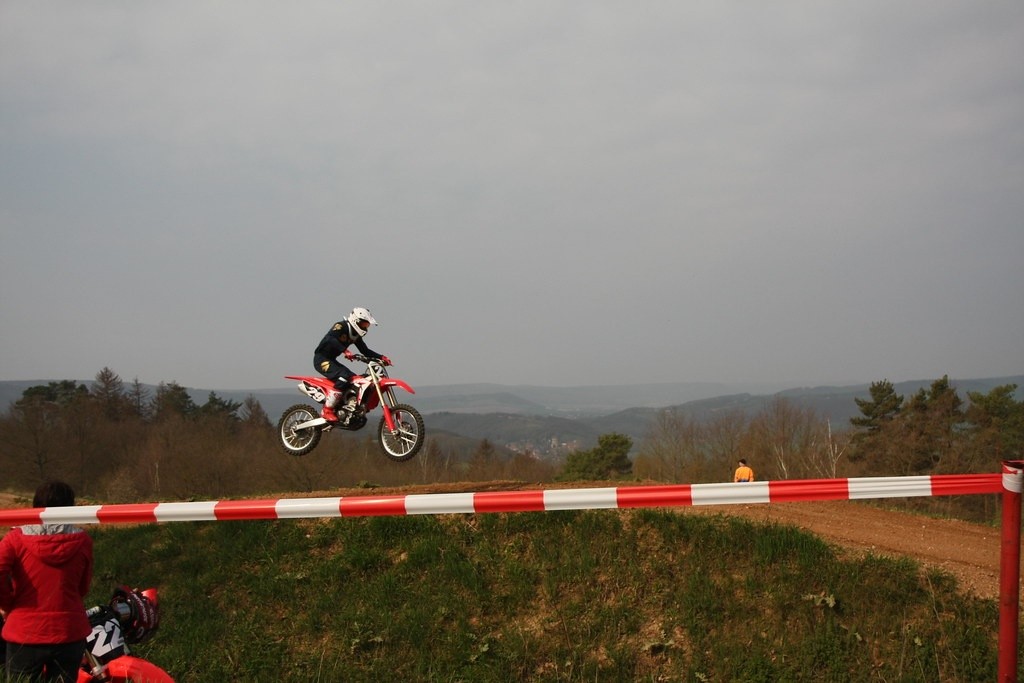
[110,585,159,645]
[349,307,378,336]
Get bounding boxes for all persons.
[313,308,393,421]
[0,479,94,683]
[733,459,754,482]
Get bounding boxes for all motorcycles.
[276,354,425,462]
[43,586,177,683]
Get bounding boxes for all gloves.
[343,350,354,361]
[381,355,392,367]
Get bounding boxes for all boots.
[320,388,343,423]
